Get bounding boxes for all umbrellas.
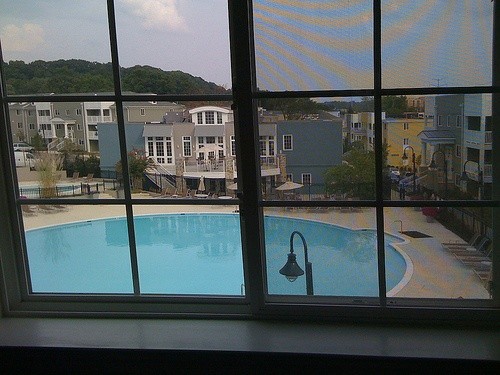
[276,181,304,199]
[422,207,438,216]
[227,182,237,190]
[195,144,224,164]
[198,176,205,194]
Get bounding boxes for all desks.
[195,194,208,200]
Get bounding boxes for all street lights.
[460,158,484,238]
[279,231,313,295]
[429,150,449,217]
[402,146,416,195]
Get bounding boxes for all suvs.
[13,142,36,154]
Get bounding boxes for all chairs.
[309,197,330,210]
[152,188,197,200]
[335,197,361,212]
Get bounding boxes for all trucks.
[14,152,43,171]
